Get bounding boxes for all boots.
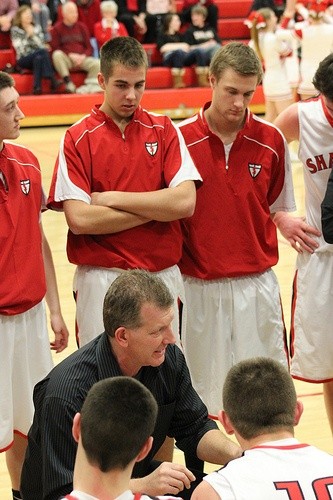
[171,68,187,88]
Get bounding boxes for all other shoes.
[66,83,75,92]
[32,87,42,95]
[51,80,64,93]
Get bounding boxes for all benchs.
[0,0,265,126]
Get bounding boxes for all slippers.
[195,66,210,86]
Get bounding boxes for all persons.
[45,36,204,463]
[20,268,243,500]
[177,43,297,472]
[0,71,69,500]
[190,352,333,500]
[273,52,333,433]
[60,376,158,500]
[0,0,333,123]
[190,357,333,500]
